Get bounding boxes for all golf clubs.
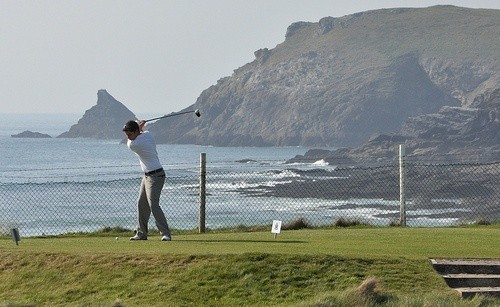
[145,109,201,123]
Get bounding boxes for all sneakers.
[161,235,170,241]
[130,234,147,240]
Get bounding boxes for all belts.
[145,168,163,176]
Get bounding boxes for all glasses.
[123,125,131,129]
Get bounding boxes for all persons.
[123,120,172,241]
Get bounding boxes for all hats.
[122,121,138,131]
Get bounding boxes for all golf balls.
[116,237,119,240]
